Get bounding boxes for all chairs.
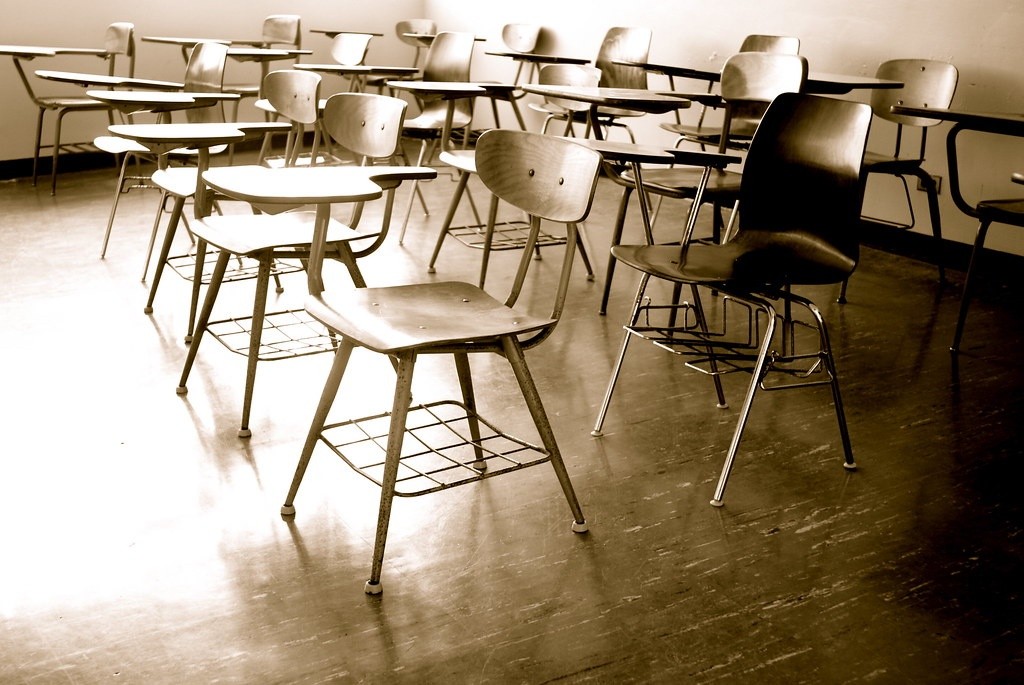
[526,25,658,216]
[428,65,602,287]
[834,59,958,308]
[946,125,1024,363]
[281,130,604,595]
[426,23,542,174]
[180,15,302,166]
[398,31,475,246]
[593,93,874,507]
[176,92,408,438]
[336,19,436,150]
[601,52,809,347]
[100,42,229,283]
[145,70,322,344]
[652,33,802,226]
[256,31,371,166]
[32,21,143,197]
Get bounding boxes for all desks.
[0,0,1024,599]
